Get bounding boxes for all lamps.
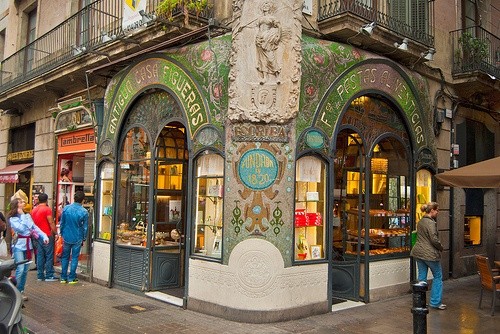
[424,47,435,63]
[139,10,180,27]
[395,38,408,50]
[349,22,375,40]
[102,32,139,44]
[71,42,110,56]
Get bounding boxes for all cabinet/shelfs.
[99,179,114,241]
[342,209,411,256]
[62,182,95,208]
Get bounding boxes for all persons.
[410,201,447,309]
[0,191,90,307]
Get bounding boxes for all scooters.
[0,257,33,334]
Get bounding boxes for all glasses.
[434,210,439,212]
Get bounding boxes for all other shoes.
[438,304,447,310]
[60,279,68,283]
[22,293,28,301]
[68,279,78,284]
[37,276,60,282]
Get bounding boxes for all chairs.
[476,255,500,316]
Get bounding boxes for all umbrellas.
[433,157,500,189]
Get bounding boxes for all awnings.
[0,163,33,184]
